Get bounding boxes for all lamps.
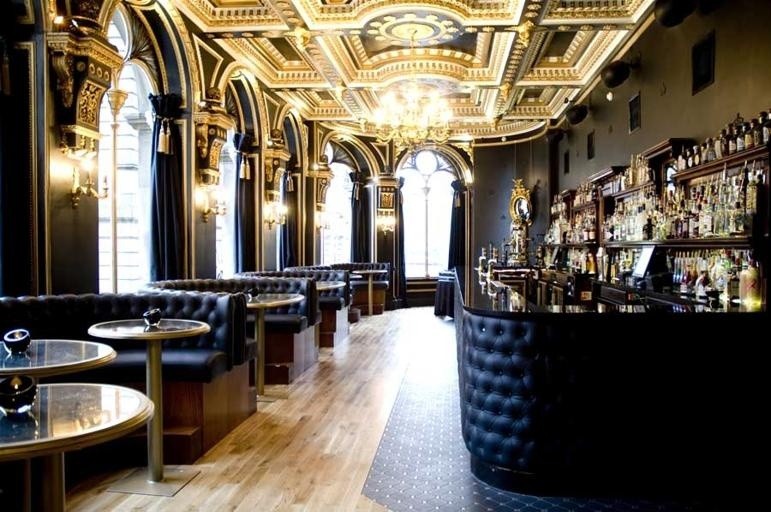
[599,56,643,89]
[564,101,596,127]
[652,2,698,27]
[366,28,459,145]
[263,201,288,231]
[66,154,112,208]
[196,184,226,222]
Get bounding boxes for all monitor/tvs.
[631,245,657,278]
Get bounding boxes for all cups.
[1,413,40,440]
[143,307,162,325]
[248,300,261,304]
[1,374,38,414]
[248,287,262,299]
[1,329,30,354]
[144,327,162,332]
[3,354,35,369]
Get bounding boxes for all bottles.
[544,182,596,276]
[675,112,771,244]
[477,272,498,310]
[486,245,497,278]
[596,153,674,240]
[604,247,638,284]
[672,306,703,314]
[478,246,487,272]
[669,247,763,306]
